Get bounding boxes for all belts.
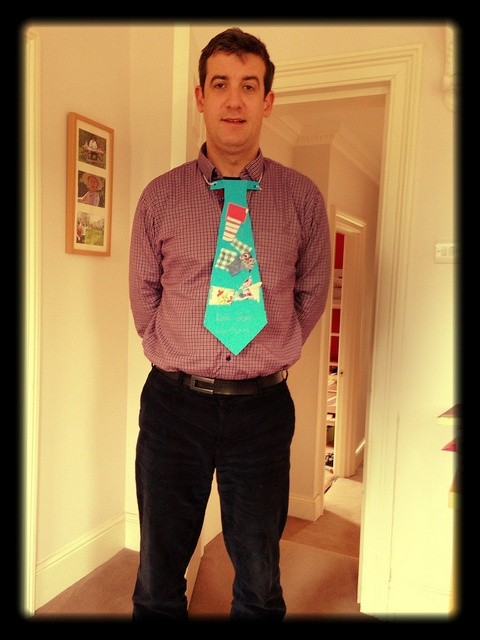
[154,365,284,394]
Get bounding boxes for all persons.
[129,27,332,619]
[78,173,105,208]
[89,136,98,160]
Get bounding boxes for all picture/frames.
[63,112,115,258]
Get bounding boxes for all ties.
[203,180,268,356]
